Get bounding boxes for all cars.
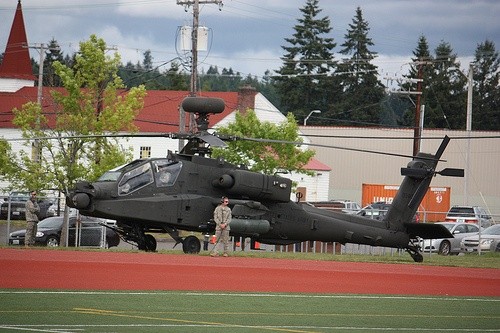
[0,191,53,221]
[458,223,500,255]
[405,222,483,255]
[8,215,120,250]
[357,202,420,223]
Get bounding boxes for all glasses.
[224,201,228,203]
[32,194,36,195]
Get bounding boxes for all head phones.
[221,196,225,203]
[29,193,33,200]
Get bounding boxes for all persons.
[210,197,232,257]
[24,191,41,246]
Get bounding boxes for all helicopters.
[0,94,468,263]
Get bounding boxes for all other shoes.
[210,252,219,256]
[223,253,228,256]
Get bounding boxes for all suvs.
[332,199,360,214]
[446,204,492,228]
